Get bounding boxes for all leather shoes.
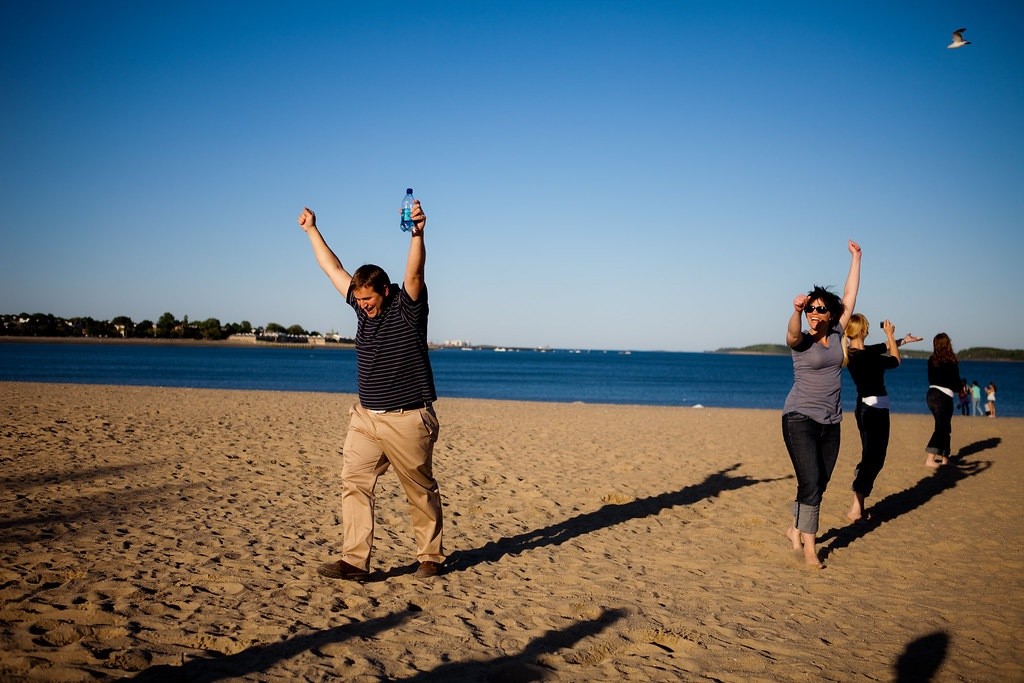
[317,560,369,581]
[415,561,449,578]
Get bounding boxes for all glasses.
[804,305,831,313]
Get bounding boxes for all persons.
[782,240,996,568]
[299,200,445,580]
[925,333,963,467]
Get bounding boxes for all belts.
[370,400,432,413]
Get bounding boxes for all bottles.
[400,188,416,232]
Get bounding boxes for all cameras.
[880,322,884,328]
